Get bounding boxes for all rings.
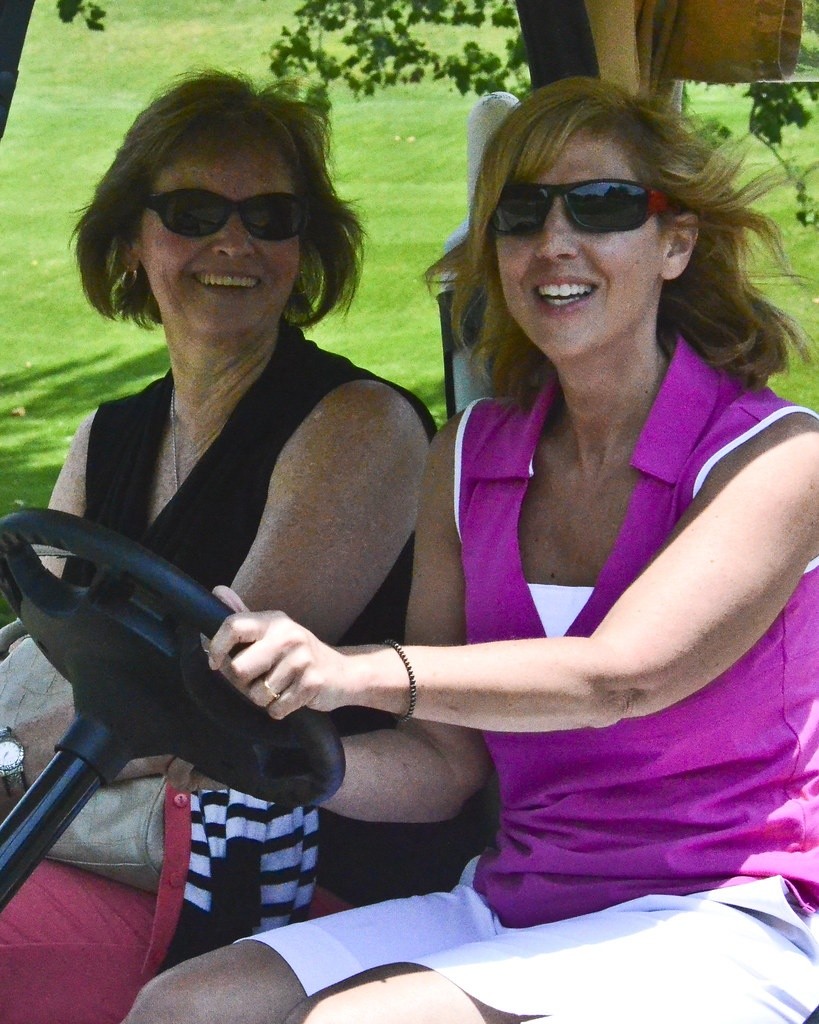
[262,677,280,700]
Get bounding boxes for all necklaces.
[171,387,179,493]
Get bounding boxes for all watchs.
[0,727,26,800]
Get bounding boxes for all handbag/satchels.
[1,616,168,894]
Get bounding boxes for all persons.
[199,73,819,1024]
[0,68,503,1024]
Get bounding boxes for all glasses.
[144,188,309,241]
[490,178,682,234]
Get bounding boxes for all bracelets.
[383,638,418,722]
